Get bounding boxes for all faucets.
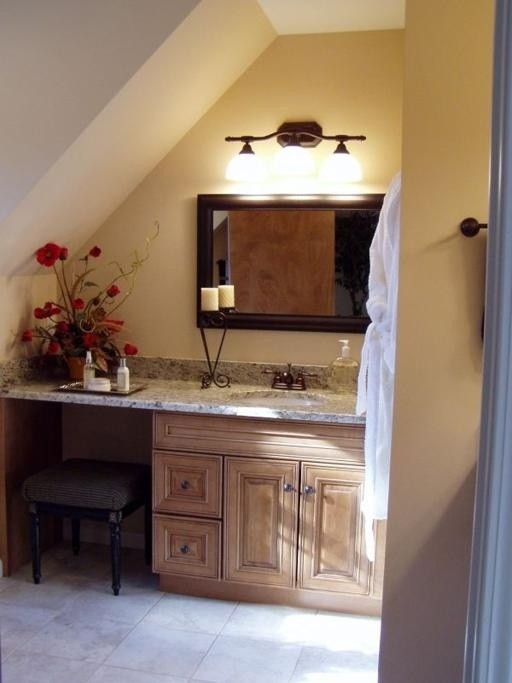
[283,371,294,384]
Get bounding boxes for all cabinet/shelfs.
[149,410,387,616]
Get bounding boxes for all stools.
[22,457,152,596]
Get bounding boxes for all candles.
[200,287,219,310]
[217,284,235,307]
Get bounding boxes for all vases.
[64,356,86,379]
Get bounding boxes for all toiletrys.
[327,339,361,391]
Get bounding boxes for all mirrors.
[193,192,386,333]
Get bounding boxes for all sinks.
[228,391,330,407]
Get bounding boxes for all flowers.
[20,220,161,372]
